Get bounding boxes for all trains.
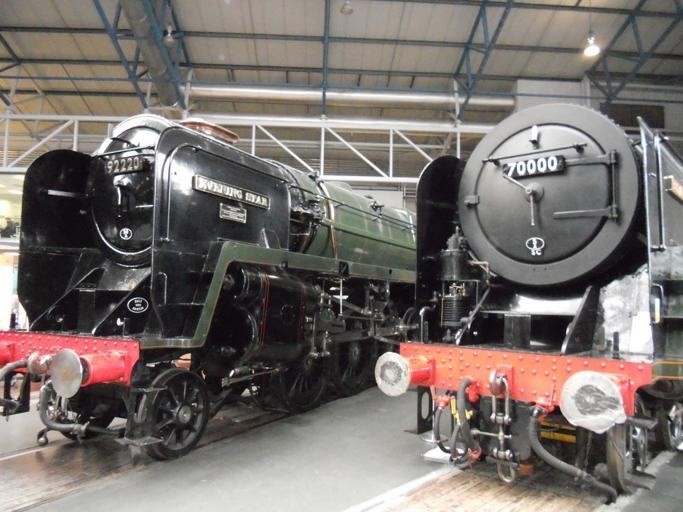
[1,112,419,466]
[372,103,683,503]
